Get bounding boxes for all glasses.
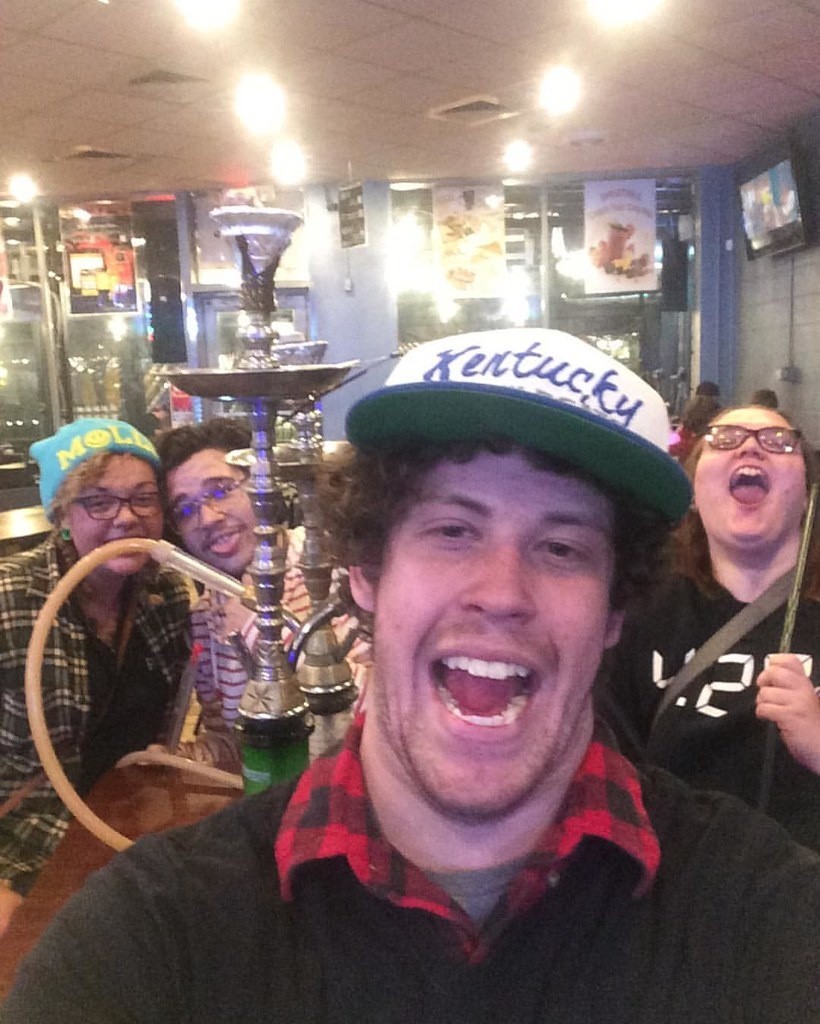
[68,481,167,520]
[167,475,250,536]
[695,425,803,454]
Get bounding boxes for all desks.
[0,759,256,1024]
[0,506,55,555]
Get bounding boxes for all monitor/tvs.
[734,140,814,261]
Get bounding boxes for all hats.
[346,325,691,526]
[30,420,165,519]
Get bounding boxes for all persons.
[148,420,372,773]
[0,418,191,894]
[753,390,778,407]
[670,382,722,454]
[0,329,820,1024]
[129,390,170,437]
[599,405,820,849]
